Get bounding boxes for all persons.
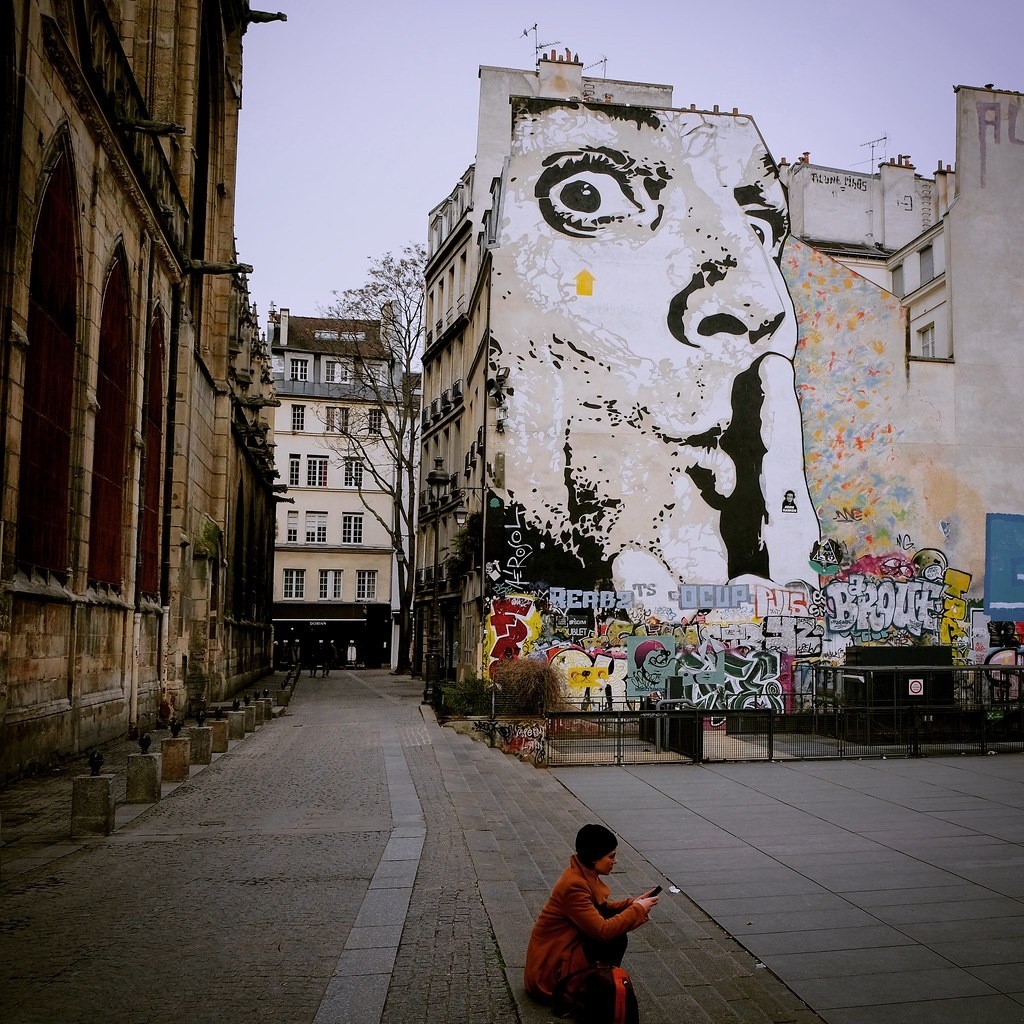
[306,645,334,679]
[497,646,518,669]
[524,823,659,1018]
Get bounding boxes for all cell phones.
[648,885,663,898]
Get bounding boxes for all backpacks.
[550,959,639,1024]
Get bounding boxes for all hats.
[575,824,618,862]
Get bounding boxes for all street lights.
[423,457,451,703]
